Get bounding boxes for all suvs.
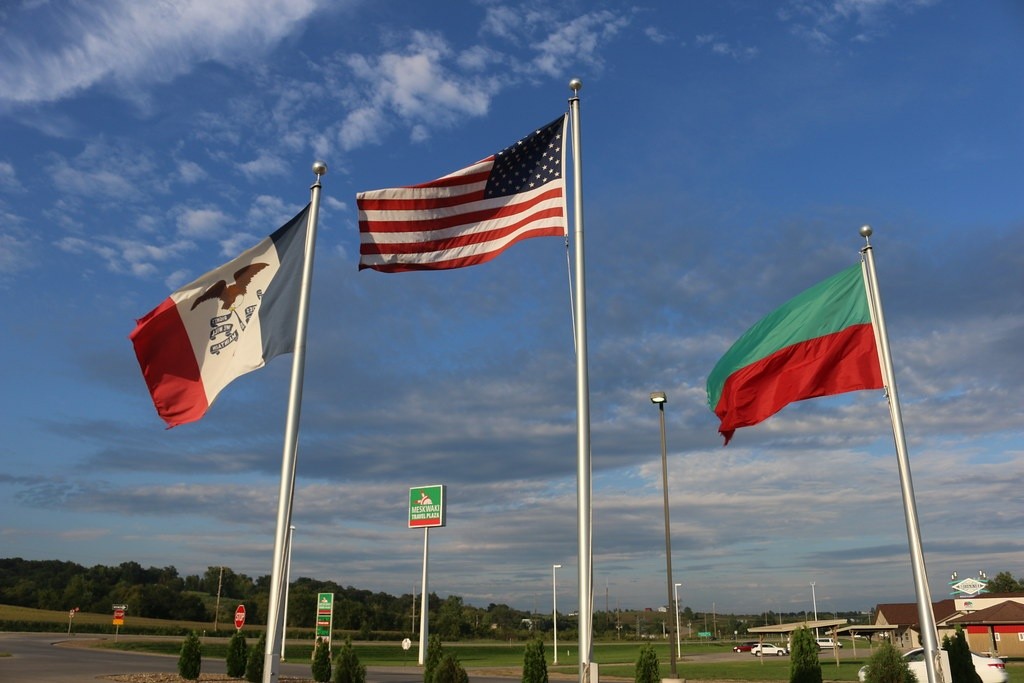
[751,643,786,657]
[733,640,761,653]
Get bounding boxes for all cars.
[787,640,821,653]
[857,646,1008,683]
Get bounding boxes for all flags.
[705,259,889,446]
[355,114,586,274]
[129,198,313,430]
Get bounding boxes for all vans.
[815,637,843,650]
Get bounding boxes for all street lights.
[648,391,679,678]
[674,583,682,661]
[552,564,562,667]
[280,525,296,661]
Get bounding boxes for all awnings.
[747,619,847,667]
[825,624,898,656]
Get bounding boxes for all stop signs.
[114,609,123,619]
[235,605,245,630]
